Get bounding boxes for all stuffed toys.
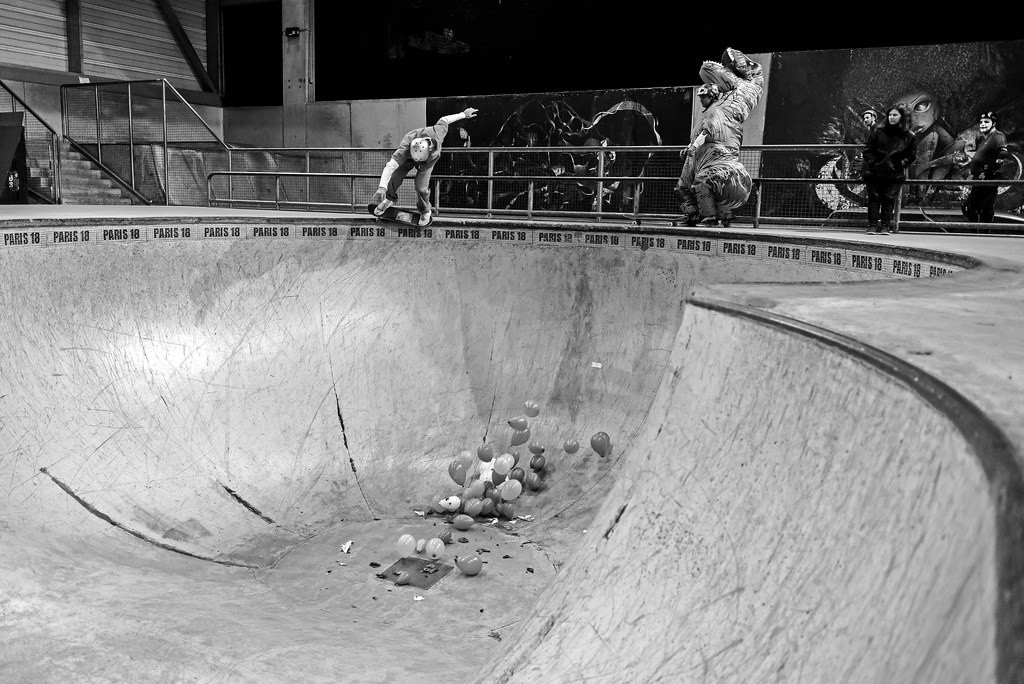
[672,46,765,231]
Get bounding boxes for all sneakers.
[418,210,432,226]
[374,198,393,216]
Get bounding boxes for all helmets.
[697,81,719,97]
[862,109,878,118]
[410,138,429,162]
[981,111,999,122]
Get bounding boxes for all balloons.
[396,570,412,586]
[456,551,483,576]
[437,527,453,544]
[425,537,446,560]
[520,399,541,418]
[597,431,611,449]
[563,437,580,455]
[416,537,428,553]
[590,434,609,459]
[506,417,529,432]
[439,440,547,528]
[509,426,531,447]
[396,533,416,559]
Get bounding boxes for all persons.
[964,110,1014,234]
[862,101,918,237]
[862,109,880,133]
[679,82,720,162]
[370,106,479,228]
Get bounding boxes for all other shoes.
[968,211,995,235]
[866,225,891,235]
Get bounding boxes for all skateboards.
[621,214,687,226]
[367,203,433,228]
